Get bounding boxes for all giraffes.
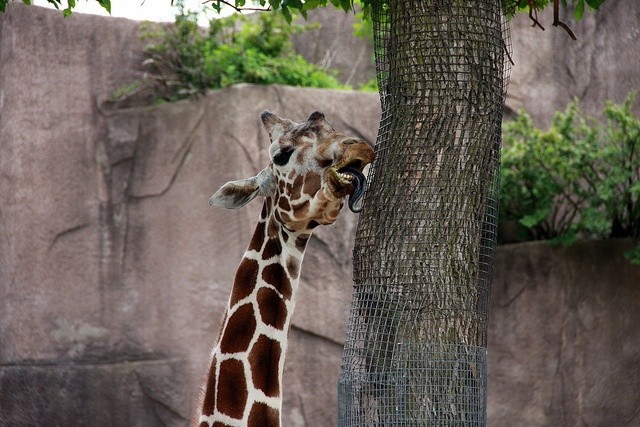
[191,109,376,427]
